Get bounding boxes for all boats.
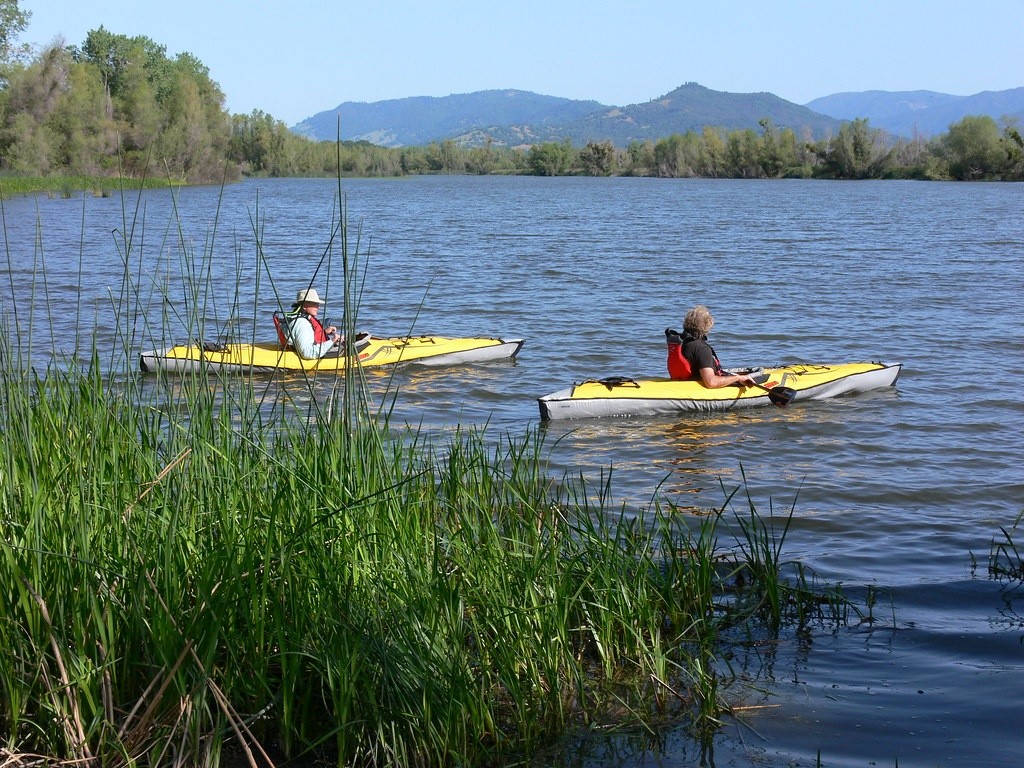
[142,328,526,377]
[539,360,907,420]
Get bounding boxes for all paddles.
[323,314,351,352]
[721,371,797,408]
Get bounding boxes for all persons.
[681,305,756,389]
[273,289,344,360]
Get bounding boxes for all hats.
[297,288,327,304]
